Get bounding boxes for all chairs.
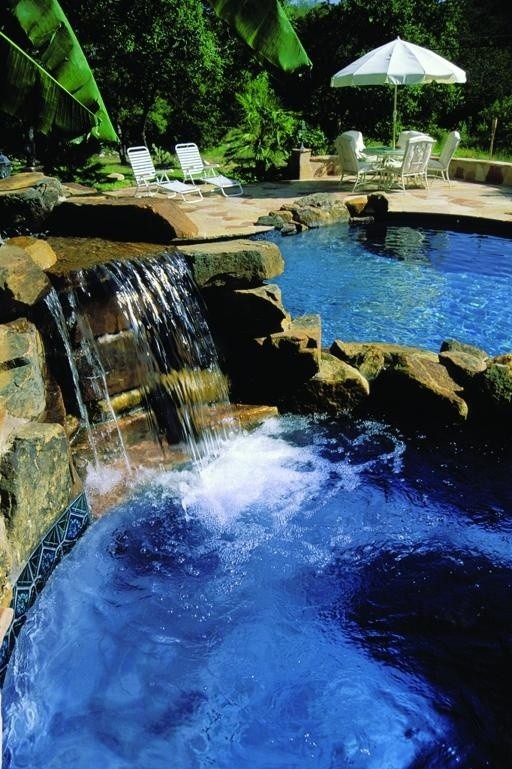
[334,129,460,195]
[127,142,244,203]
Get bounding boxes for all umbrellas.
[330,33,469,151]
